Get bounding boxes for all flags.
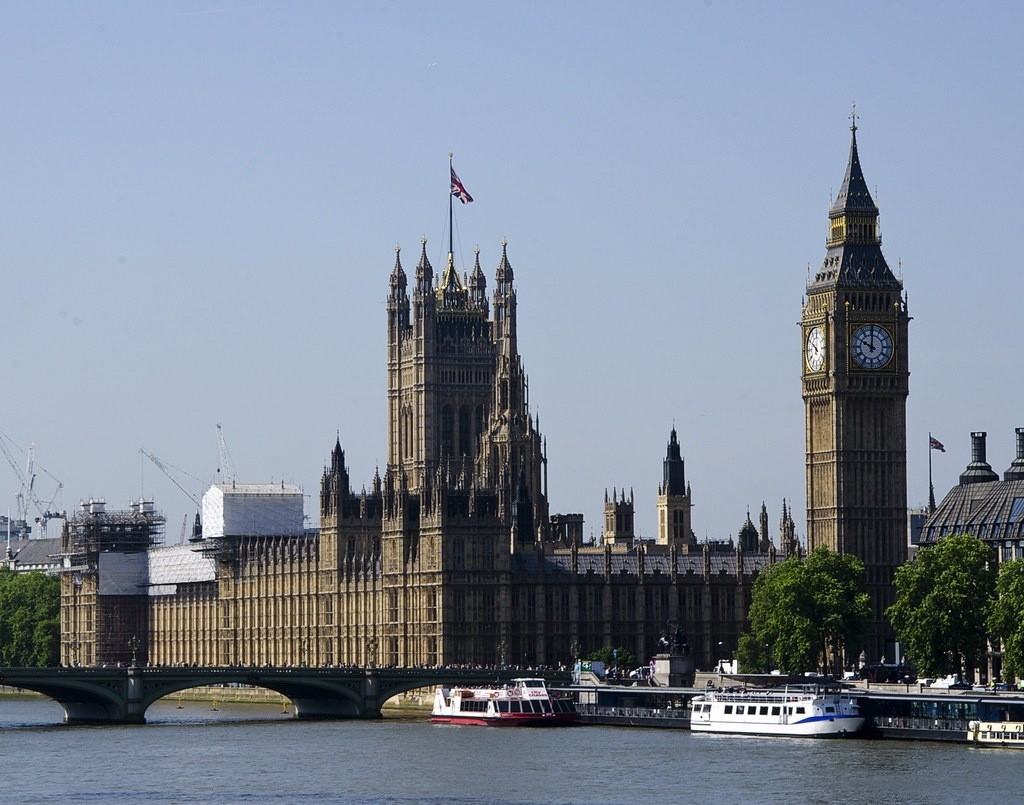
[450,166,474,205]
[930,436,946,452]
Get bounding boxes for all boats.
[689,685,864,738]
[430,677,556,728]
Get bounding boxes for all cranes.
[0,430,63,539]
[138,422,239,509]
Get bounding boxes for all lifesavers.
[506,690,513,696]
[514,689,520,695]
[494,692,499,698]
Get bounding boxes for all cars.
[949,684,973,690]
[630,667,651,679]
[990,683,1018,692]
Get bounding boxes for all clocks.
[850,324,892,369]
[808,328,824,371]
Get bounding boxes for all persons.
[604,665,631,678]
[146,661,306,672]
[460,684,499,690]
[321,662,571,672]
